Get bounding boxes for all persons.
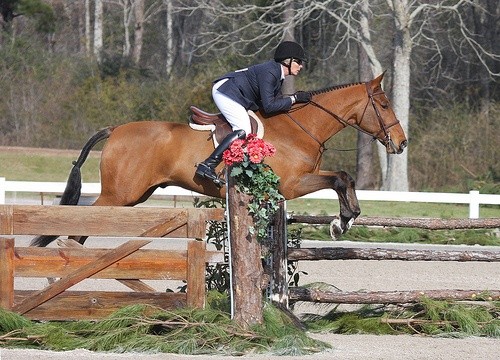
[196,41,312,184]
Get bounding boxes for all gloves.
[293,91,312,102]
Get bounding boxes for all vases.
[226,166,289,327]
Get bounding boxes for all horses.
[29,67,409,287]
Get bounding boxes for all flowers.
[218,132,286,240]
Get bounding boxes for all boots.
[195,129,246,185]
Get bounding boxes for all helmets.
[274,41,309,64]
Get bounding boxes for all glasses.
[293,59,302,65]
